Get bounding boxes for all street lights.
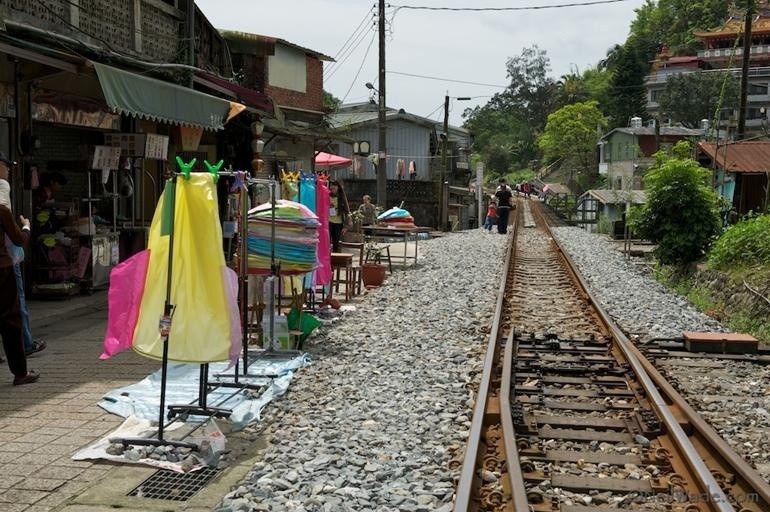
[365,82,385,210]
[438,133,448,228]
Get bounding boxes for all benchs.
[365,244,393,275]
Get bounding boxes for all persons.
[483,202,499,234]
[524,181,531,199]
[328,181,353,253]
[516,182,521,194]
[0,203,41,387]
[495,183,512,234]
[495,178,515,206]
[0,151,48,357]
[358,194,378,245]
[90,205,110,225]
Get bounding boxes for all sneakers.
[14,370,40,385]
[24,338,46,356]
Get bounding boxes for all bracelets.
[21,225,31,232]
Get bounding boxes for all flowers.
[364,241,382,265]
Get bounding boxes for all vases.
[361,263,385,286]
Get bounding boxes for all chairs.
[336,240,364,296]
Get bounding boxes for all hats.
[0,153,17,165]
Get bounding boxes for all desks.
[360,227,432,270]
[330,252,354,302]
